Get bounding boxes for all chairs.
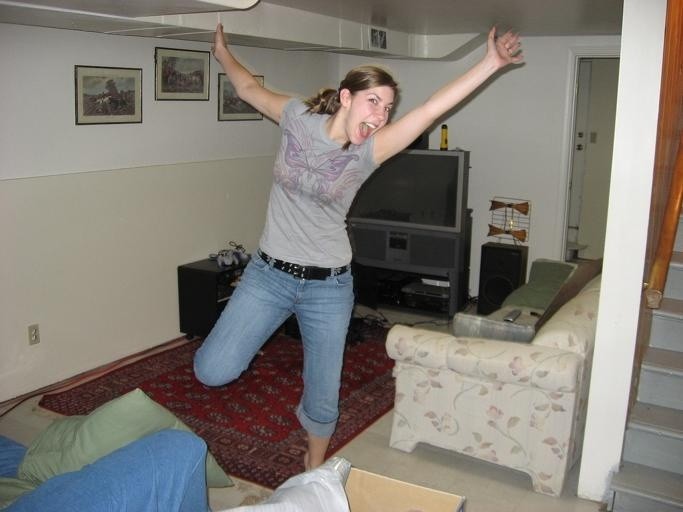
[74,46,265,126]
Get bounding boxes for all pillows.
[0,386,235,512]
[450,311,536,345]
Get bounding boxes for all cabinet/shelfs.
[177,253,252,341]
[344,208,473,322]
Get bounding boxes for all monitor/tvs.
[347,149,470,234]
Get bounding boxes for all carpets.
[35,324,396,490]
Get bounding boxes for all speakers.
[476,241,529,316]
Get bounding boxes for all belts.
[257,247,352,280]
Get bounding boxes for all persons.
[193,20,526,470]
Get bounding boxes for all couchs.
[384,258,602,496]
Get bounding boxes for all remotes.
[503,309,522,323]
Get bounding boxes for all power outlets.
[27,323,41,346]
